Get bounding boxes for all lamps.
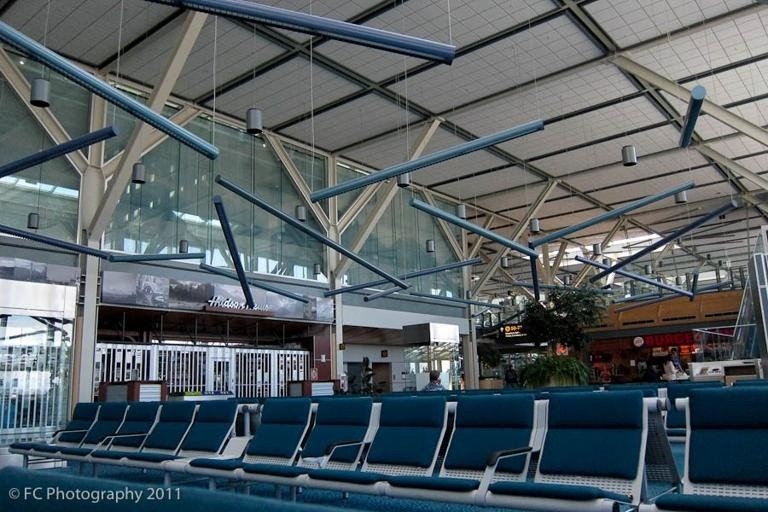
[397,2,687,268]
[26,0,306,256]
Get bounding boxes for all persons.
[662,347,685,374]
[422,370,448,391]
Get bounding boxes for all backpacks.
[507,371,515,379]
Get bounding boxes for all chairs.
[0,378,768,512]
